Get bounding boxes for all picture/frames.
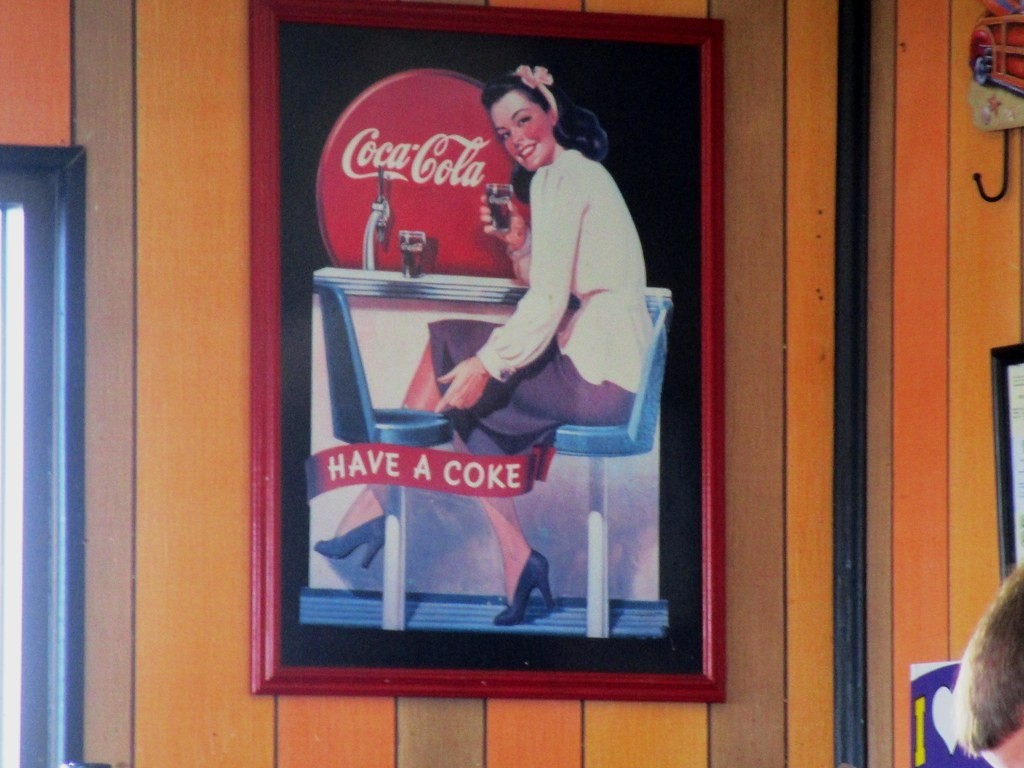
[246,1,726,703]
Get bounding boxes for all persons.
[952,564,1024,768]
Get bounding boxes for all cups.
[398,231,427,278]
[488,184,513,232]
